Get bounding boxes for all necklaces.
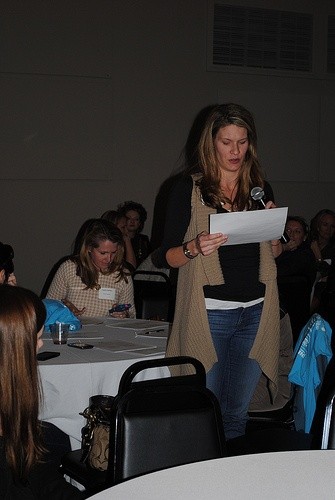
[223,180,237,205]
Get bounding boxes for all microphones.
[251,187,290,244]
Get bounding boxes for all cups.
[49,323,70,345]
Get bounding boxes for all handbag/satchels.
[77,394,117,472]
[279,307,294,357]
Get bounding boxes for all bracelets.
[182,241,198,261]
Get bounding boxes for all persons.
[0,241,17,286]
[0,284,83,500]
[276,209,335,338]
[100,200,150,278]
[44,219,137,321]
[150,103,285,441]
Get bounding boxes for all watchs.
[317,259,321,261]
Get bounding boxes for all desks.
[86,450,335,500]
[36,318,173,491]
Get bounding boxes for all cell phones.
[68,343,94,349]
[109,303,131,313]
[36,351,60,361]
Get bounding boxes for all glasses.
[286,226,305,235]
[0,242,15,270]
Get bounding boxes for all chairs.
[61,356,228,500]
[133,271,175,322]
[285,311,335,450]
